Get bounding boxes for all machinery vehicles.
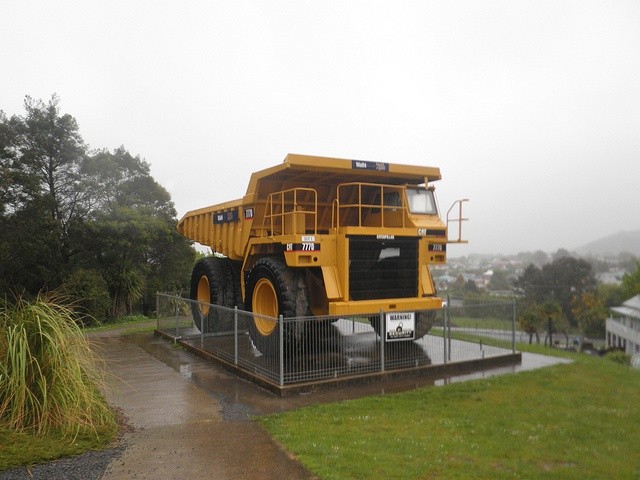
[176,153,468,357]
